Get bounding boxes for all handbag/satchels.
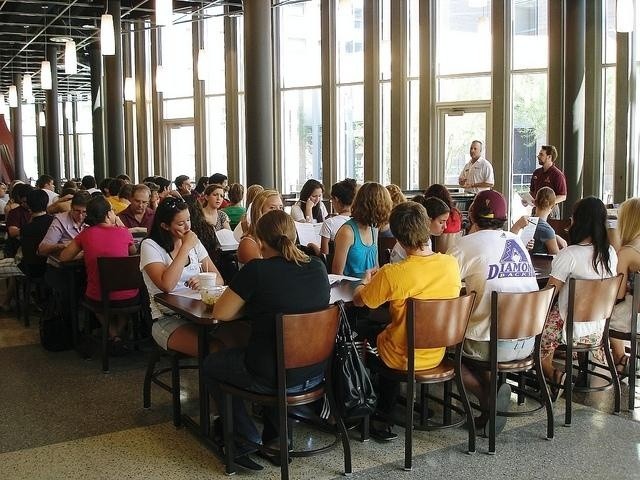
[40,320,73,352]
[327,342,377,417]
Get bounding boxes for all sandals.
[111,335,129,356]
[551,368,567,401]
[611,355,629,383]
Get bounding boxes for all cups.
[198,272,217,299]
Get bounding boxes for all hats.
[472,189,507,219]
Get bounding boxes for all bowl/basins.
[200,287,224,306]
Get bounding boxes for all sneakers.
[232,437,262,458]
[474,383,511,438]
[263,429,291,456]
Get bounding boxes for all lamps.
[0,0,213,128]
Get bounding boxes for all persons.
[541,196,618,403]
[520,146,567,220]
[608,195,638,380]
[1,172,568,412]
[447,190,539,437]
[353,201,461,440]
[213,210,332,457]
[458,140,495,208]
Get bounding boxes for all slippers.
[369,420,398,441]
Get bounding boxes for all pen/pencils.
[527,220,536,226]
[299,200,307,204]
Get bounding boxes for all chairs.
[443,283,557,456]
[1,191,639,373]
[208,300,353,480]
[360,290,477,473]
[609,268,639,413]
[518,271,623,428]
[137,266,201,430]
